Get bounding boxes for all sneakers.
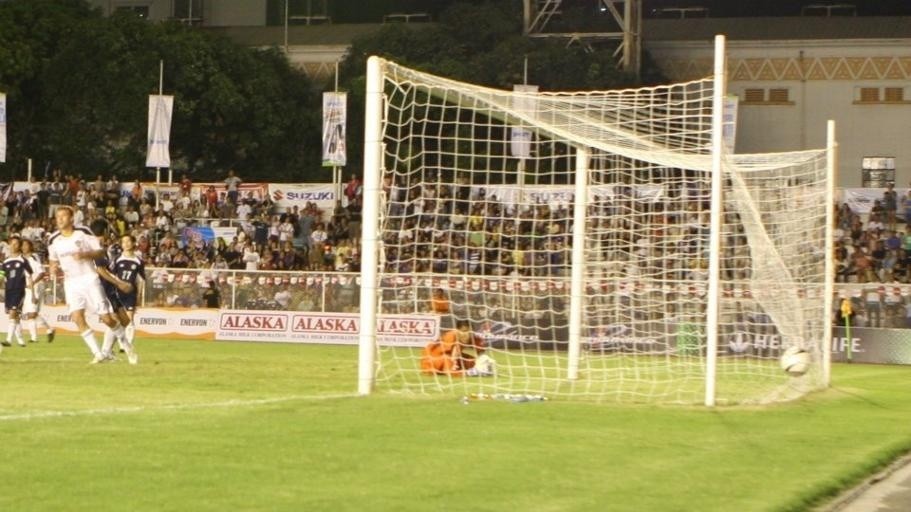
[20,340,39,347]
[47,328,56,342]
[0,340,11,346]
[91,343,139,364]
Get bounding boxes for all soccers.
[781,343,813,376]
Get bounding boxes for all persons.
[421,320,496,379]
[2,158,363,366]
[378,171,911,328]
